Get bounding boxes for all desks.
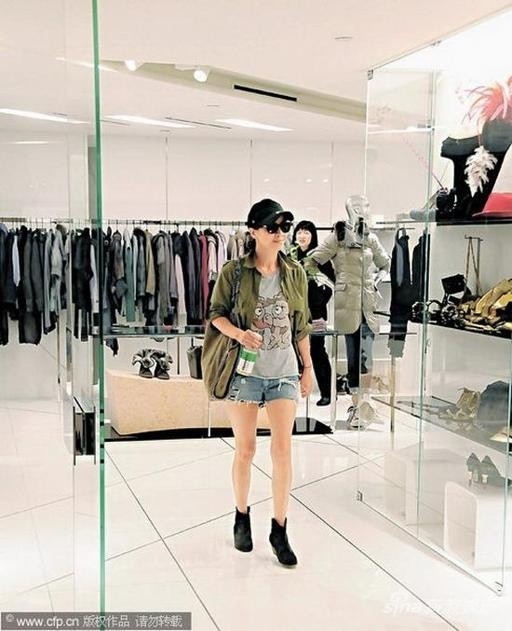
[67,324,419,439]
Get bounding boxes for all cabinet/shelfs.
[358,3,511,599]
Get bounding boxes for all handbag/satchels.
[186,313,242,400]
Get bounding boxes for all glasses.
[259,220,292,234]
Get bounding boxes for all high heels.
[233,507,253,552]
[467,453,511,488]
[268,517,298,565]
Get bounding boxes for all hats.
[247,198,294,229]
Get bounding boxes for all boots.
[132,349,173,379]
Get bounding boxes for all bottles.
[234,328,263,381]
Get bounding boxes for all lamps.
[194,65,212,82]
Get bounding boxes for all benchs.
[105,368,271,435]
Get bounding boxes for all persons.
[284,220,336,405]
[306,195,391,429]
[210,199,314,566]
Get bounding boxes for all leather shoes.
[316,397,330,406]
